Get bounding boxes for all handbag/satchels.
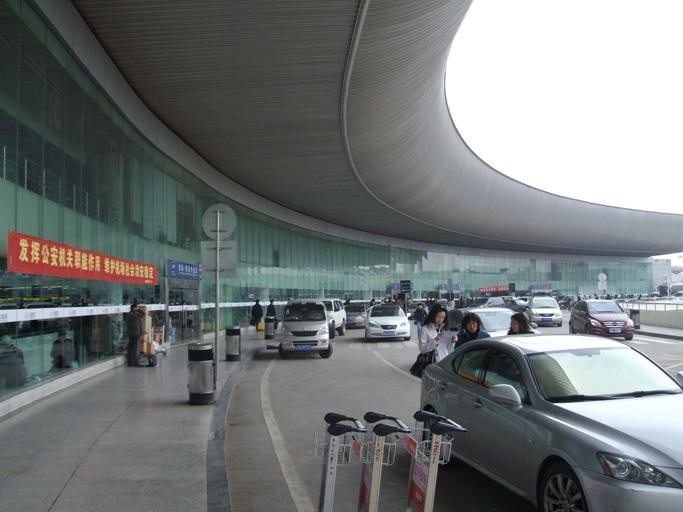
[409,351,436,378]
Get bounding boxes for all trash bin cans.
[225,325,242,361]
[264,316,274,339]
[187,341,216,405]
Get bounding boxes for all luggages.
[257,322,264,331]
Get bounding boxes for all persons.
[413,295,491,363]
[252,299,276,323]
[509,313,533,335]
[125,303,147,368]
[568,293,642,309]
[370,296,412,311]
[49,330,76,369]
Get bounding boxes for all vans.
[278,300,333,359]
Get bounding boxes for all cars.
[365,305,410,341]
[506,300,527,308]
[569,299,633,340]
[421,335,681,511]
[345,304,368,329]
[447,307,542,337]
[466,296,506,307]
[529,296,562,327]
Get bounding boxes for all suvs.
[322,298,346,338]
